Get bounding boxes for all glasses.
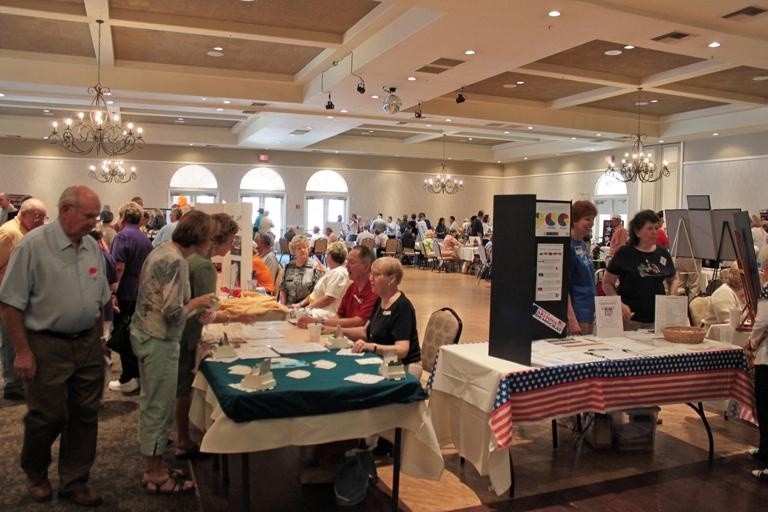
[369,271,395,277]
[207,234,216,242]
[33,212,50,223]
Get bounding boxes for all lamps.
[43,19,145,184]
[382,86,402,115]
[421,134,464,195]
[414,100,422,118]
[604,87,670,184]
[456,87,465,104]
[350,51,365,93]
[321,71,334,110]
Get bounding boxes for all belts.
[27,327,99,341]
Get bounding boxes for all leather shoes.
[751,468,768,481]
[745,448,760,458]
[56,482,105,507]
[25,473,54,504]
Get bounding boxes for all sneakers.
[4,390,27,400]
[107,376,138,393]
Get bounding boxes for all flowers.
[221,286,242,300]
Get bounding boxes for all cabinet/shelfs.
[196,203,252,292]
[603,219,624,247]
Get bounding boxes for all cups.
[382,349,399,364]
[246,278,258,292]
[307,322,323,343]
[729,309,741,330]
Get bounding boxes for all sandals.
[141,467,183,486]
[145,476,195,494]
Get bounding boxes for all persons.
[562,201,768,479]
[0,183,493,506]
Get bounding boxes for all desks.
[702,319,758,412]
[424,327,762,501]
[187,318,446,512]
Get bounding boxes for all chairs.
[310,238,328,269]
[278,238,291,264]
[361,236,492,285]
[594,269,606,288]
[421,306,463,400]
[689,296,715,337]
